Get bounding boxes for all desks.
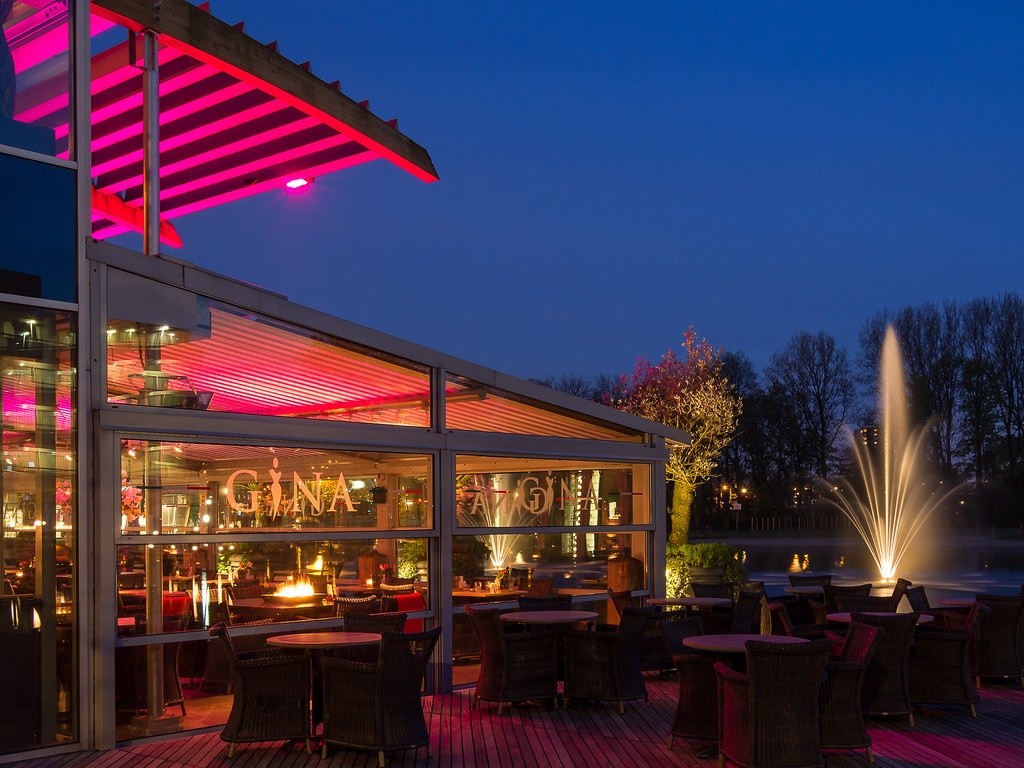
[646,597,731,677]
[119,588,184,635]
[826,612,934,625]
[785,585,824,598]
[499,611,601,706]
[940,599,975,606]
[230,599,334,613]
[265,633,382,750]
[681,634,810,759]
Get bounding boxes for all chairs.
[0,576,1024,768]
